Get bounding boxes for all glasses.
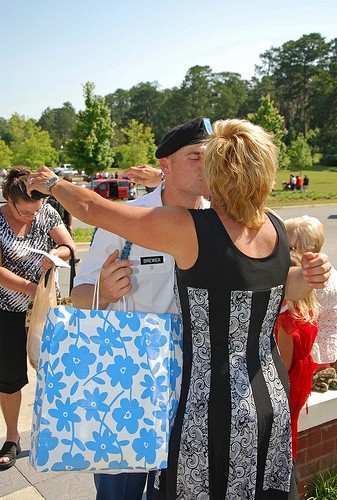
[12,202,43,217]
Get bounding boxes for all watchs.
[45,176,62,195]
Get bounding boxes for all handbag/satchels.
[26,268,57,373]
[29,268,183,475]
[26,244,76,376]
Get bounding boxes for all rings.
[29,178,33,185]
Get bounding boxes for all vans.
[88,176,136,201]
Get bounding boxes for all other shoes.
[70,258,80,264]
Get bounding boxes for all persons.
[127,182,137,201]
[273,214,337,500]
[283,174,309,192]
[71,116,332,500]
[96,170,118,179]
[46,175,73,249]
[0,166,77,468]
[26,118,293,500]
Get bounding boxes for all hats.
[155,116,213,159]
[49,167,65,179]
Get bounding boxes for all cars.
[54,163,79,177]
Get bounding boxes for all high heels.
[0,437,21,467]
